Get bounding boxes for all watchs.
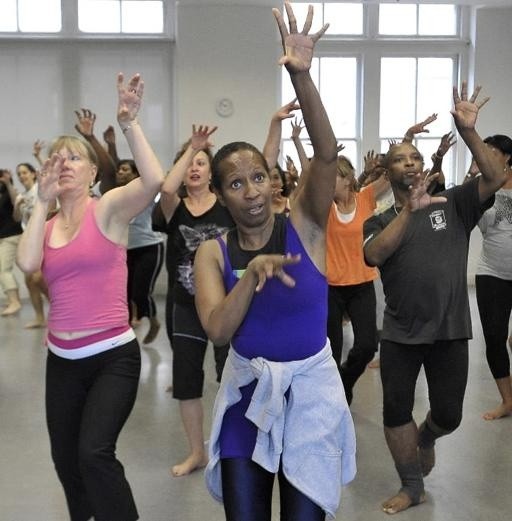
[466,173,474,178]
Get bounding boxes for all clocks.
[216,97,234,116]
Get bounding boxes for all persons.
[14,71,165,521]
[71,107,117,199]
[13,139,49,330]
[309,113,439,407]
[0,168,24,317]
[354,275,399,370]
[267,117,309,219]
[462,135,512,421]
[426,130,458,195]
[194,0,357,521]
[349,137,401,187]
[103,126,166,345]
[361,80,509,516]
[152,98,304,479]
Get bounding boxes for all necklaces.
[55,214,79,231]
[391,203,401,217]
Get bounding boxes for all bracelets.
[123,122,139,132]
[362,171,368,178]
[435,154,443,160]
